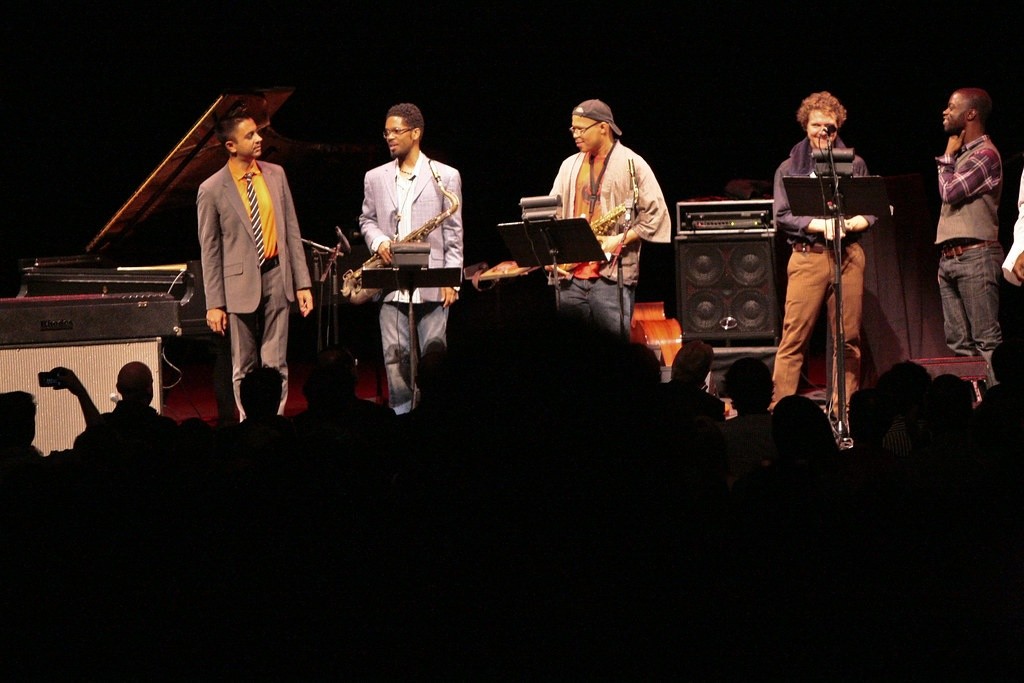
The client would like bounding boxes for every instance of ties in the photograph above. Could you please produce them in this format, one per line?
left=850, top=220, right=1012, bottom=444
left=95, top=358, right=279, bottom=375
left=244, top=171, right=266, bottom=269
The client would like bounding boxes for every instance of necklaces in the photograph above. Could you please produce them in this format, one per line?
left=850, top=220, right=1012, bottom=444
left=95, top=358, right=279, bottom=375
left=588, top=141, right=617, bottom=223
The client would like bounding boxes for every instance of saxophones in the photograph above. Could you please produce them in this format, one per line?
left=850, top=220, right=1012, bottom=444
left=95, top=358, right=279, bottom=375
left=543, top=158, right=639, bottom=274
left=339, top=173, right=460, bottom=307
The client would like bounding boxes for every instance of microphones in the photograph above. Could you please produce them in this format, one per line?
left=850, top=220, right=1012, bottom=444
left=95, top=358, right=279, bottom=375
left=822, top=125, right=837, bottom=136
left=625, top=190, right=635, bottom=222
left=335, top=225, right=354, bottom=254
left=109, top=393, right=120, bottom=404
left=0, top=336, right=163, bottom=457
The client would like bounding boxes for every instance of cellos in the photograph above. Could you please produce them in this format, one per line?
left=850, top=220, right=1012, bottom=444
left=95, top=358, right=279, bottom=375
left=629, top=300, right=731, bottom=418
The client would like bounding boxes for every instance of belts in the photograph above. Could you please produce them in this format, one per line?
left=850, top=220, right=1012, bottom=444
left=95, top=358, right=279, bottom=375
left=260, top=257, right=279, bottom=274
left=940, top=239, right=995, bottom=257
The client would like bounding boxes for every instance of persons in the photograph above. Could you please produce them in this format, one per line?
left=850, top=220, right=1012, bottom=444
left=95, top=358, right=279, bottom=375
left=359, top=105, right=463, bottom=415
left=218, top=340, right=472, bottom=682
left=197, top=106, right=313, bottom=422
left=0, top=367, right=104, bottom=683
left=694, top=394, right=843, bottom=683
left=934, top=88, right=1003, bottom=392
left=548, top=99, right=671, bottom=341
left=660, top=338, right=775, bottom=472
left=773, top=91, right=878, bottom=446
left=73, top=360, right=307, bottom=683
left=849, top=359, right=972, bottom=453
left=1002, top=169, right=1024, bottom=287
left=973, top=344, right=1024, bottom=415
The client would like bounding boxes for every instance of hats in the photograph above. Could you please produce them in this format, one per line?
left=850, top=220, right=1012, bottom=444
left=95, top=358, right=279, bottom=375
left=574, top=98, right=622, bottom=136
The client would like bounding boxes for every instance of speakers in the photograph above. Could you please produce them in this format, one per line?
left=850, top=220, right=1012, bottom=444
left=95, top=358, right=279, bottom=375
left=674, top=234, right=778, bottom=347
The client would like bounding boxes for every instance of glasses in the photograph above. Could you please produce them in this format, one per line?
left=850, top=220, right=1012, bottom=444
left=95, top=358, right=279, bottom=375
left=383, top=128, right=414, bottom=139
left=569, top=121, right=600, bottom=134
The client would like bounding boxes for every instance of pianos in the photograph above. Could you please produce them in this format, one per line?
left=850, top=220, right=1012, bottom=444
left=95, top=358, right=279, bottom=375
left=1, top=91, right=298, bottom=345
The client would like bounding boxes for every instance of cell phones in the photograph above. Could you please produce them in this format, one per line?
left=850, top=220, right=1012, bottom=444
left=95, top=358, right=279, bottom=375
left=38, top=371, right=67, bottom=387
